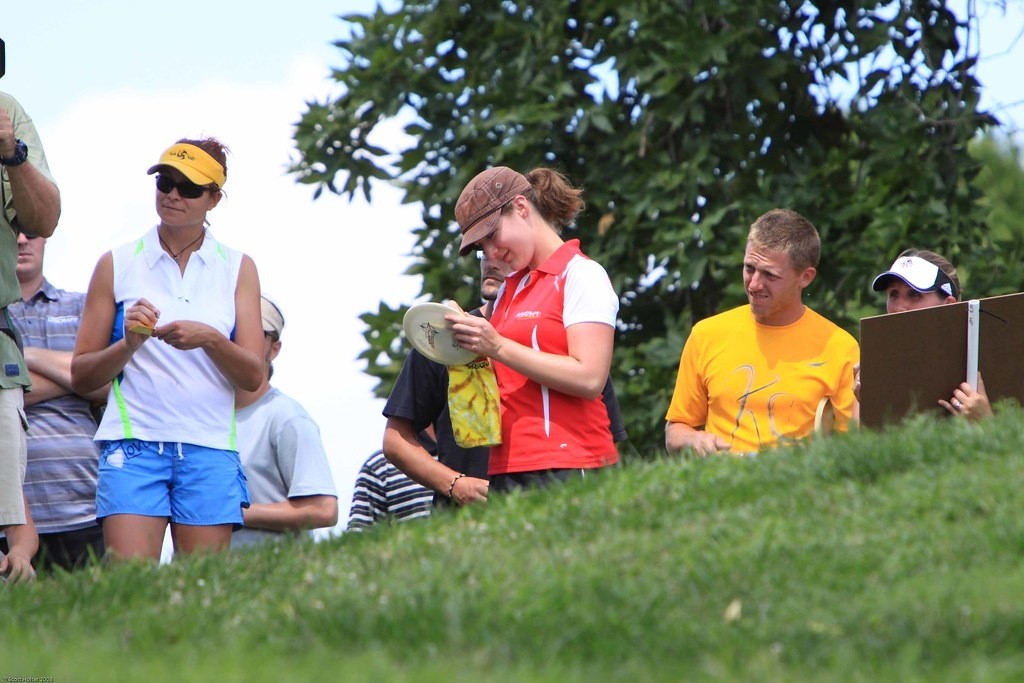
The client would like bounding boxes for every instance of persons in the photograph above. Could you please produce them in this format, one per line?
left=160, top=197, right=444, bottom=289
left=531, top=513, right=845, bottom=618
left=71, top=137, right=266, bottom=566
left=850, top=247, right=992, bottom=422
left=18, top=227, right=109, bottom=576
left=0, top=91, right=59, bottom=589
left=445, top=165, right=621, bottom=496
left=383, top=225, right=631, bottom=522
left=347, top=420, right=439, bottom=530
left=664, top=207, right=862, bottom=458
left=228, top=298, right=340, bottom=550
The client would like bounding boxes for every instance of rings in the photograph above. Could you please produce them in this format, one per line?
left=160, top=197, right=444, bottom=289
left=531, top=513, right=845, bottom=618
left=953, top=401, right=962, bottom=410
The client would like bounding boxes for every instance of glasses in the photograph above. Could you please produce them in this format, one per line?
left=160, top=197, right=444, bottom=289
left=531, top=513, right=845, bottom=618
left=476, top=251, right=487, bottom=259
left=155, top=175, right=215, bottom=198
left=9, top=223, right=38, bottom=239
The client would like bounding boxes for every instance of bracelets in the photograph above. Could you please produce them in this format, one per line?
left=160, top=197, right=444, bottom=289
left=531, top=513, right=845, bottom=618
left=448, top=473, right=468, bottom=500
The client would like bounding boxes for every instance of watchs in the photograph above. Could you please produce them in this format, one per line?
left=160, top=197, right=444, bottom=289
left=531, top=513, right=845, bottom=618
left=1, top=138, right=29, bottom=166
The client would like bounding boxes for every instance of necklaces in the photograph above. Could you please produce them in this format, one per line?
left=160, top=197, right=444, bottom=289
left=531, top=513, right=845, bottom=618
left=157, top=228, right=205, bottom=265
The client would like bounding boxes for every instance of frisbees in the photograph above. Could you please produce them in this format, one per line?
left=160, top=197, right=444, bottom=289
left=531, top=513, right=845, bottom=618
left=403, top=303, right=480, bottom=366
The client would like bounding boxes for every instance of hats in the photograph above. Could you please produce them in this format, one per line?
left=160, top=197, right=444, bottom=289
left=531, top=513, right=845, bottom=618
left=455, top=166, right=532, bottom=256
left=260, top=296, right=284, bottom=339
left=148, top=142, right=226, bottom=191
left=873, top=255, right=957, bottom=298
